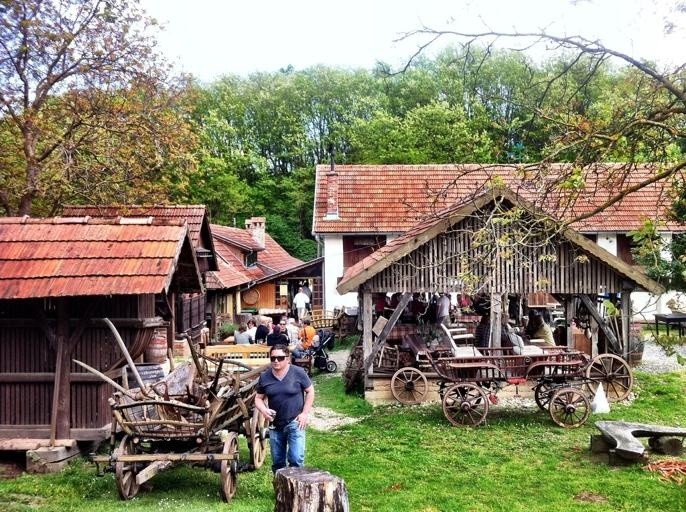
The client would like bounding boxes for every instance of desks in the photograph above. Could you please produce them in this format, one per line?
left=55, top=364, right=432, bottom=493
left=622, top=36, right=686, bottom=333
left=224, top=336, right=236, bottom=344
left=654, top=312, right=686, bottom=337
left=194, top=357, right=271, bottom=372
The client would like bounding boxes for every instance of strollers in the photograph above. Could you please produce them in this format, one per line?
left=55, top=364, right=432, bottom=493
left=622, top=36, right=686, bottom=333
left=297, top=327, right=337, bottom=376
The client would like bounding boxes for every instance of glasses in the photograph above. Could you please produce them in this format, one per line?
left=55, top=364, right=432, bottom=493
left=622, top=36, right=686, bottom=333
left=271, top=356, right=289, bottom=361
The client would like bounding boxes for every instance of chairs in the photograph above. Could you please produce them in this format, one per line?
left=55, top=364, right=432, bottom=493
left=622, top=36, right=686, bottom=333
left=404, top=324, right=549, bottom=368
left=294, top=344, right=314, bottom=376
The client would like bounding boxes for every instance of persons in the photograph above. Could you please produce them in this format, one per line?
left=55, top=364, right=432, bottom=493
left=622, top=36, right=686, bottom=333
left=376, top=292, right=451, bottom=324
left=234, top=318, right=320, bottom=357
left=293, top=284, right=312, bottom=321
left=476, top=312, right=508, bottom=348
left=256, top=344, right=315, bottom=474
left=526, top=310, right=556, bottom=346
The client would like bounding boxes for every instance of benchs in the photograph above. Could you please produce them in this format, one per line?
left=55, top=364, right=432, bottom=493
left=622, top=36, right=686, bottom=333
left=308, top=307, right=337, bottom=329
left=593, top=420, right=685, bottom=464
left=202, top=344, right=270, bottom=359
